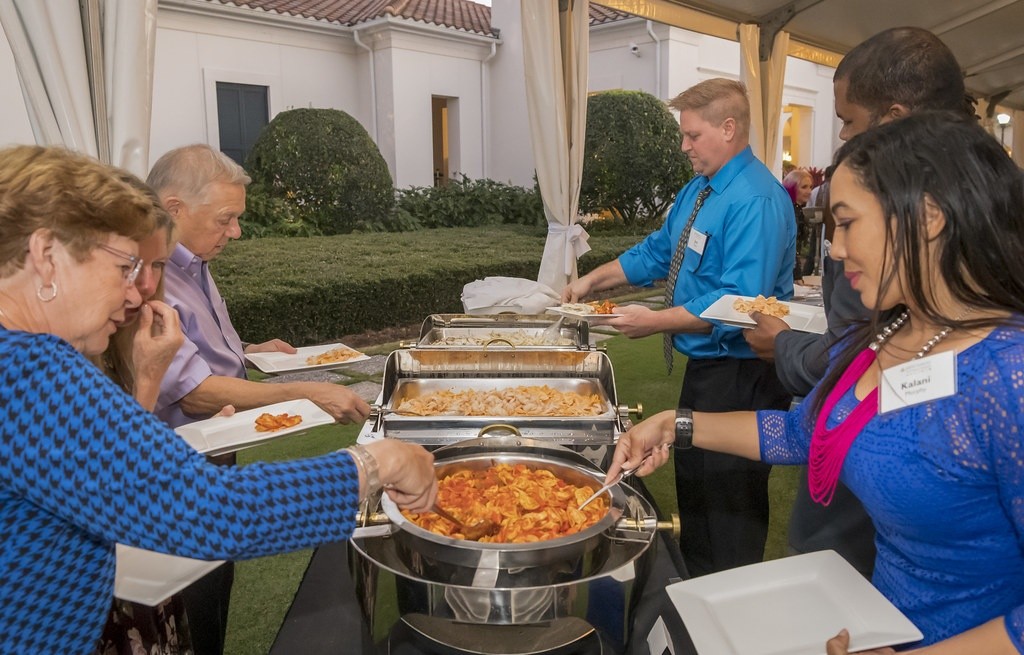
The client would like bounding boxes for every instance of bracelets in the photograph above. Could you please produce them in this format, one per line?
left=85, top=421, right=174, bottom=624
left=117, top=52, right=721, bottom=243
left=336, top=444, right=383, bottom=526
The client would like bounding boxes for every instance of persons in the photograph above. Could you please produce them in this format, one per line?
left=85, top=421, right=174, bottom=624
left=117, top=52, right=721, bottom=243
left=83, top=143, right=372, bottom=655
left=0, top=145, right=439, bottom=655
left=742, top=25, right=967, bottom=397
left=561, top=77, right=797, bottom=578
left=604, top=111, right=1024, bottom=655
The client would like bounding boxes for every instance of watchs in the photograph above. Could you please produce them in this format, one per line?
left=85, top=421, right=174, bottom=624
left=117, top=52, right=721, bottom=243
left=674, top=409, right=694, bottom=451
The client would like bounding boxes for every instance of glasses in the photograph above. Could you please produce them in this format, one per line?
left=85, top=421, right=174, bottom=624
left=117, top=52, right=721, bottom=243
left=26, top=227, right=144, bottom=287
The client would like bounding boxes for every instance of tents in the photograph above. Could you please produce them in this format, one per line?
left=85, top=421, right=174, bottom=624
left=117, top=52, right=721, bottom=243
left=0, top=1, right=1024, bottom=313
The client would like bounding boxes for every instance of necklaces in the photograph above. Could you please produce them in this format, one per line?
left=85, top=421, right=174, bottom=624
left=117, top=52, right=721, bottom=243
left=808, top=308, right=976, bottom=506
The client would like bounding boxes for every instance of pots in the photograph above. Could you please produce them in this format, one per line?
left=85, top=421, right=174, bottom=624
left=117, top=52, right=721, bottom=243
left=347, top=422, right=681, bottom=654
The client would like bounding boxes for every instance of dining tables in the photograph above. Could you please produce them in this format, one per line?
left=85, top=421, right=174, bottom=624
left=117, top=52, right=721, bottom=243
left=269, top=447, right=693, bottom=655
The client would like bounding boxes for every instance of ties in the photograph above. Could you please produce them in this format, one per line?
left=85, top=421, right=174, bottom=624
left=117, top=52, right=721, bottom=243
left=663, top=184, right=712, bottom=375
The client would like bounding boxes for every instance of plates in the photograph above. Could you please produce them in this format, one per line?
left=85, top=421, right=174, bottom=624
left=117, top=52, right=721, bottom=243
left=698, top=294, right=828, bottom=335
left=172, top=398, right=336, bottom=456
left=665, top=549, right=924, bottom=655
left=546, top=306, right=624, bottom=322
left=244, top=342, right=372, bottom=376
left=113, top=542, right=226, bottom=607
